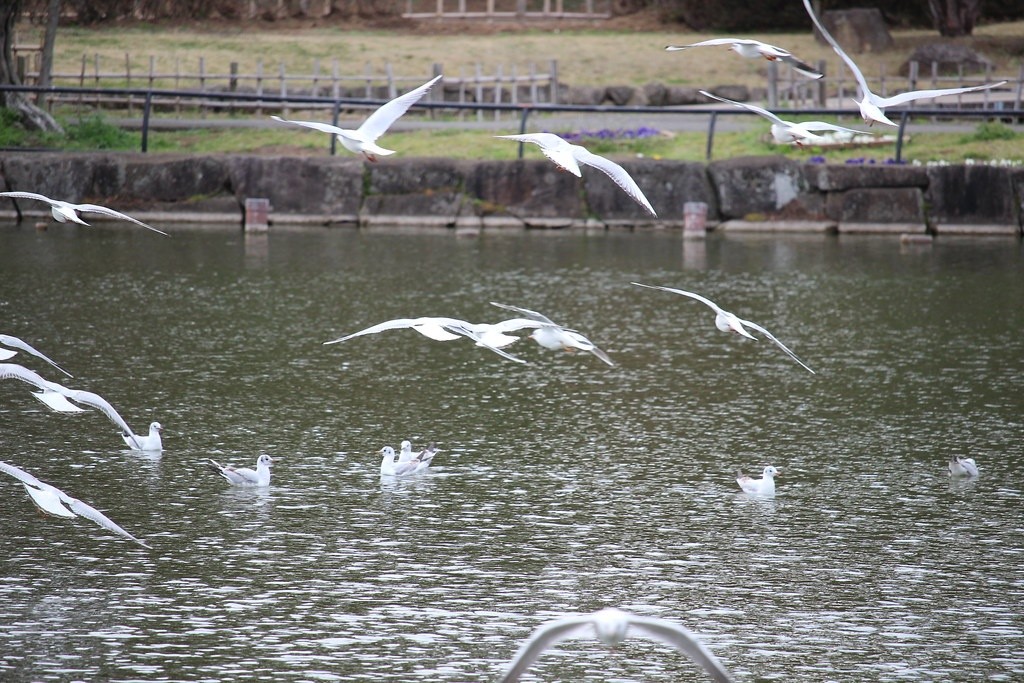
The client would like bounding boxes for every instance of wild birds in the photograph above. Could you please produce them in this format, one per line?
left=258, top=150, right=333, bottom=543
left=0, top=191, right=173, bottom=239
left=205, top=454, right=280, bottom=489
left=663, top=1, right=1009, bottom=149
left=494, top=607, right=742, bottom=683
left=269, top=75, right=444, bottom=164
left=374, top=440, right=437, bottom=477
left=0, top=332, right=164, bottom=549
left=321, top=301, right=615, bottom=374
left=491, top=132, right=659, bottom=221
left=948, top=456, right=978, bottom=478
left=630, top=282, right=816, bottom=376
left=734, top=465, right=781, bottom=498
